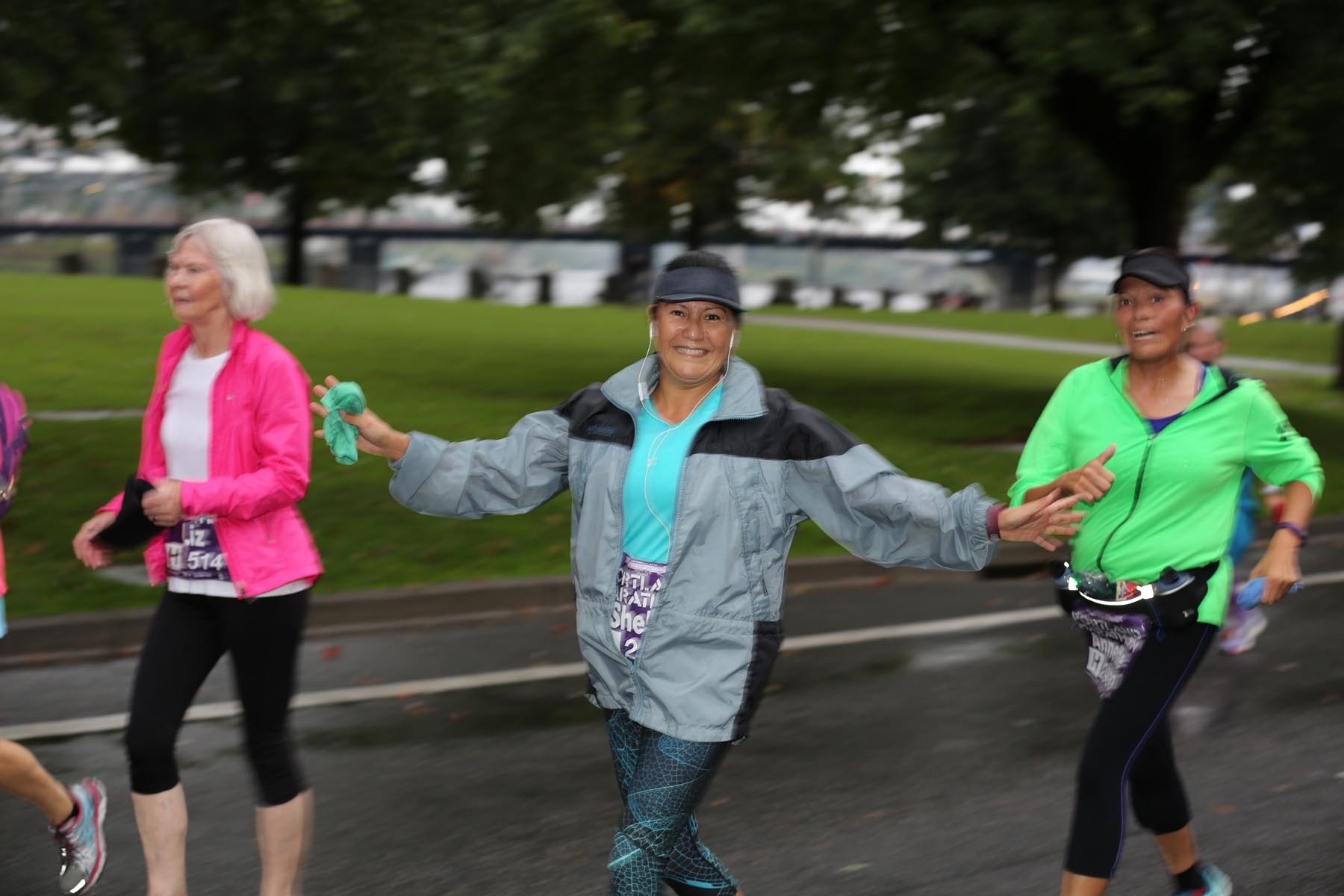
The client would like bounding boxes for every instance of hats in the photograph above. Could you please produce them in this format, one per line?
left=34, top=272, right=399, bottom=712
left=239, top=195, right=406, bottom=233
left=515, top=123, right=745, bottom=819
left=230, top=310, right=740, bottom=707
left=654, top=265, right=749, bottom=315
left=1106, top=251, right=1191, bottom=296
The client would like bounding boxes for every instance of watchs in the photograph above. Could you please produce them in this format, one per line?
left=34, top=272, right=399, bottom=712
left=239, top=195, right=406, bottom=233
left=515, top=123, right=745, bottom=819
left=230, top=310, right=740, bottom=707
left=1275, top=522, right=1309, bottom=549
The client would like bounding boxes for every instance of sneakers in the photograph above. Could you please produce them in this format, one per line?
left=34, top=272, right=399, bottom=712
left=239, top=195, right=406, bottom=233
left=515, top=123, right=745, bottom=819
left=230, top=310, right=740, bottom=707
left=48, top=775, right=109, bottom=896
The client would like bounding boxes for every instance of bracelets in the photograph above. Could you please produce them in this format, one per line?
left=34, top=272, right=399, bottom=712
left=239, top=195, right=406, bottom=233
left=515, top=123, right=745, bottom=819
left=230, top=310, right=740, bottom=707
left=986, top=502, right=1007, bottom=543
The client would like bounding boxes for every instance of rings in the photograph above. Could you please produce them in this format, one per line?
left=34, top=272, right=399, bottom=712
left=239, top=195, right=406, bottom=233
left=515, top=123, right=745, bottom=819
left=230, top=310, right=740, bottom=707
left=152, top=515, right=157, bottom=522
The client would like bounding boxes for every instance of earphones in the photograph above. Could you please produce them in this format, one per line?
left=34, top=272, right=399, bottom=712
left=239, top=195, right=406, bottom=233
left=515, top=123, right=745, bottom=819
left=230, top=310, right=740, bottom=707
left=730, top=332, right=735, bottom=348
left=649, top=322, right=655, bottom=340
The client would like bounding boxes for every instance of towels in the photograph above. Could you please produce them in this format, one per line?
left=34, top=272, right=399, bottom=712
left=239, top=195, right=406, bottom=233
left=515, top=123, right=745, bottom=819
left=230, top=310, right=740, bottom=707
left=318, top=379, right=366, bottom=465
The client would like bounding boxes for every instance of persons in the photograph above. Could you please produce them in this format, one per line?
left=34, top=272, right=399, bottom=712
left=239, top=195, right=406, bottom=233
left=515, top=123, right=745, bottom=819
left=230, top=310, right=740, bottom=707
left=71, top=219, right=324, bottom=896
left=1006, top=237, right=1327, bottom=896
left=309, top=249, right=1086, bottom=896
left=0, top=383, right=108, bottom=896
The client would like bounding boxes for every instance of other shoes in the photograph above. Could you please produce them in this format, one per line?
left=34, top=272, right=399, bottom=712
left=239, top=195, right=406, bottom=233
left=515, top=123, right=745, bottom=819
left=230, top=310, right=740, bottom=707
left=1218, top=602, right=1269, bottom=654
left=1172, top=863, right=1234, bottom=896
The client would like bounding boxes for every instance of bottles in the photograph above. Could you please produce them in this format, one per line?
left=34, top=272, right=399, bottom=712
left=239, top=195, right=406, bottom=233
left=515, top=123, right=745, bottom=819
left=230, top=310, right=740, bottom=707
left=1048, top=558, right=1075, bottom=587
left=1158, top=568, right=1192, bottom=592
